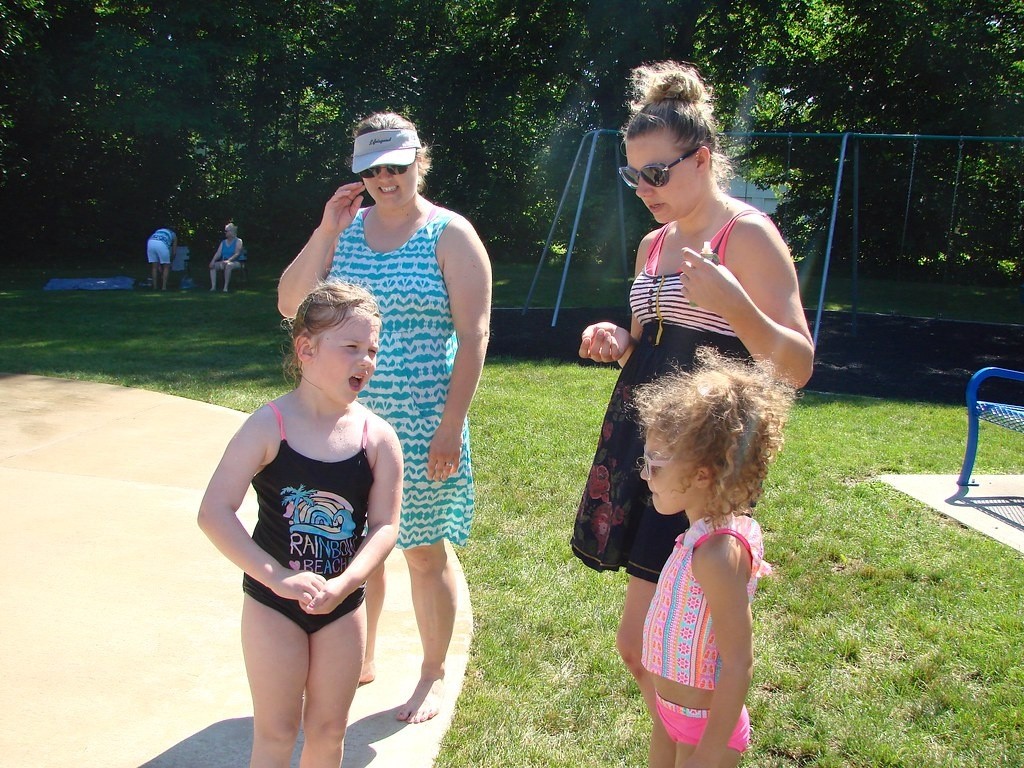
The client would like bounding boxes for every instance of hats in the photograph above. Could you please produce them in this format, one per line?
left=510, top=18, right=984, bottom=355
left=351, top=128, right=422, bottom=174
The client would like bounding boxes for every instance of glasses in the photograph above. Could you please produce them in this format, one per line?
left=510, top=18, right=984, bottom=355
left=359, top=165, right=409, bottom=178
left=617, top=145, right=713, bottom=189
left=224, top=230, right=231, bottom=234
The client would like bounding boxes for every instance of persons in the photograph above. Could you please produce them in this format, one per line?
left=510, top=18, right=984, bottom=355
left=630, top=347, right=794, bottom=768
left=148, top=227, right=178, bottom=291
left=277, top=112, right=493, bottom=723
left=197, top=281, right=405, bottom=768
left=576, top=62, right=814, bottom=723
left=208, top=222, right=243, bottom=294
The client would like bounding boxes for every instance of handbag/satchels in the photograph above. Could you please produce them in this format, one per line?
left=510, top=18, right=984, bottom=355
left=177, top=274, right=195, bottom=289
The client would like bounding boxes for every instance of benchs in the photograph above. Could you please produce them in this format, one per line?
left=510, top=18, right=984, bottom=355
left=955, top=367, right=1024, bottom=486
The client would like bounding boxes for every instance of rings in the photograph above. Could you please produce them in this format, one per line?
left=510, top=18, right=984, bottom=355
left=446, top=463, right=453, bottom=466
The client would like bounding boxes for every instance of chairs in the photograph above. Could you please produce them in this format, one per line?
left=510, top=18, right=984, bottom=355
left=215, top=247, right=247, bottom=293
